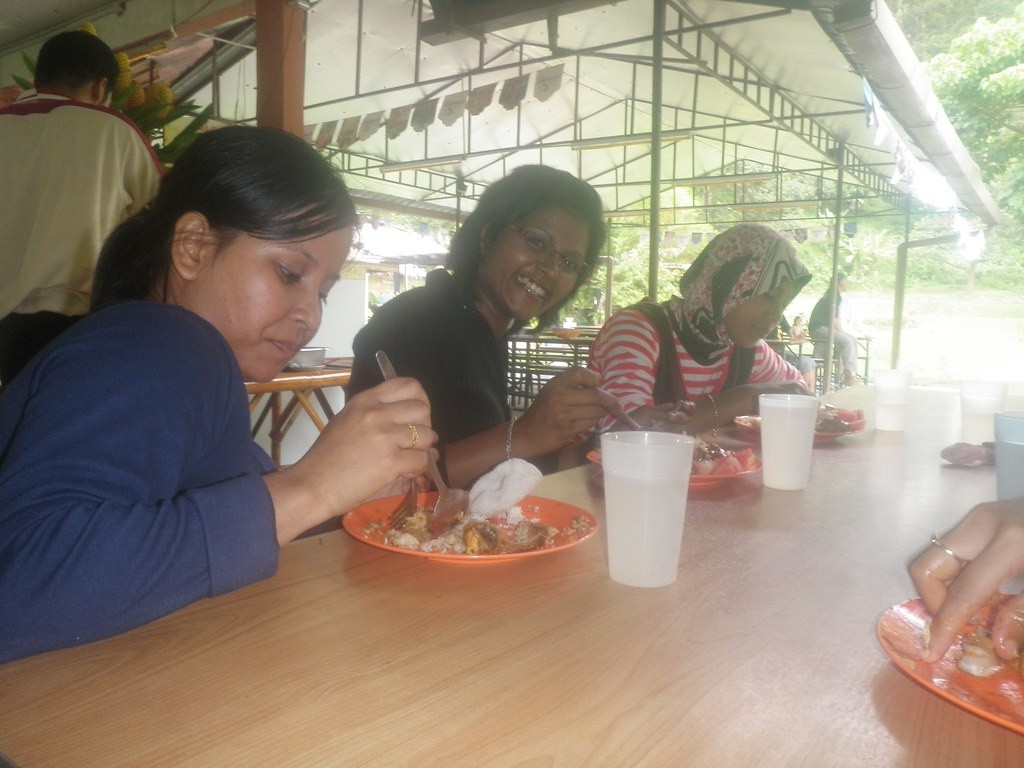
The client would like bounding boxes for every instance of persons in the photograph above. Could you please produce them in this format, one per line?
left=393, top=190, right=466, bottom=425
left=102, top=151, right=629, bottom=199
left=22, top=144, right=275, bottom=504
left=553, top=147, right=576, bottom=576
left=907, top=495, right=1024, bottom=664
left=564, top=224, right=812, bottom=464
left=1, top=29, right=167, bottom=395
left=808, top=271, right=862, bottom=386
left=766, top=315, right=819, bottom=393
left=344, top=164, right=621, bottom=493
left=0, top=126, right=440, bottom=666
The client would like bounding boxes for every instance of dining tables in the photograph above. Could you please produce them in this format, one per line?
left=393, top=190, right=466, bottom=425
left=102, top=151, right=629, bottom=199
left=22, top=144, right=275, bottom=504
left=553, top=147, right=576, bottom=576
left=0, top=386, right=1023, bottom=768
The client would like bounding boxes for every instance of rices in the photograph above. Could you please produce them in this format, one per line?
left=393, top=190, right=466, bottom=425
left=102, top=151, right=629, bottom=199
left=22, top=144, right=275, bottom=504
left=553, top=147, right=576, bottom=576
left=384, top=504, right=541, bottom=556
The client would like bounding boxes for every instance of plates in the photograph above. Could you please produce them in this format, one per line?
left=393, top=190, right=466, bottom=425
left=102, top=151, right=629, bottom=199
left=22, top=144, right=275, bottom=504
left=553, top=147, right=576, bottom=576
left=586, top=447, right=762, bottom=486
left=342, top=491, right=600, bottom=564
left=288, top=365, right=325, bottom=370
left=734, top=415, right=865, bottom=441
left=876, top=594, right=1024, bottom=735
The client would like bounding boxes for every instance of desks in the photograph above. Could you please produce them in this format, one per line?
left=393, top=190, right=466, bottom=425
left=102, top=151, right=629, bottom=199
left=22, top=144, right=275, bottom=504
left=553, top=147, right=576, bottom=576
left=506, top=326, right=828, bottom=410
left=243, top=357, right=353, bottom=465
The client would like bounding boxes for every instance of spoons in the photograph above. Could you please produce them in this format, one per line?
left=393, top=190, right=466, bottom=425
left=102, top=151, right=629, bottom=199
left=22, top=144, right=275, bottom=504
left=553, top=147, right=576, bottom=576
left=377, top=351, right=469, bottom=522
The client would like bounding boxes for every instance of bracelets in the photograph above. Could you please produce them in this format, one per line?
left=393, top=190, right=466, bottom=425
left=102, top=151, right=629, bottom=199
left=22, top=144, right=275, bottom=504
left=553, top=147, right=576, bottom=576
left=707, top=394, right=720, bottom=437
left=504, top=416, right=518, bottom=460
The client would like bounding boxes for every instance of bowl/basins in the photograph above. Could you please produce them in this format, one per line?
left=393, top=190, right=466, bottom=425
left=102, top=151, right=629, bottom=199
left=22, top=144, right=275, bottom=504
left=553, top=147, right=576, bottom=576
left=553, top=328, right=582, bottom=337
left=291, top=346, right=325, bottom=366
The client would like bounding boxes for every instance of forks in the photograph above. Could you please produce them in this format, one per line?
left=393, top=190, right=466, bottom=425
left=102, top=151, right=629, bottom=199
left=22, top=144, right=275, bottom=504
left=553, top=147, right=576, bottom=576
left=385, top=478, right=418, bottom=531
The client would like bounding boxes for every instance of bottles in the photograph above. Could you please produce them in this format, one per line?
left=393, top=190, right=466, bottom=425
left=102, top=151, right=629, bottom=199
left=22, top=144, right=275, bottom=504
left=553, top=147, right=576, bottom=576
left=775, top=325, right=782, bottom=340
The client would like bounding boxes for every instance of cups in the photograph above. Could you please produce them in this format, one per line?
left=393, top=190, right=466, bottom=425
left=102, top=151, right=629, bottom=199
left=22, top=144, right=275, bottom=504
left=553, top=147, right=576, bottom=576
left=994, top=412, right=1024, bottom=502
left=758, top=393, right=819, bottom=489
left=874, top=368, right=910, bottom=430
left=600, top=430, right=696, bottom=587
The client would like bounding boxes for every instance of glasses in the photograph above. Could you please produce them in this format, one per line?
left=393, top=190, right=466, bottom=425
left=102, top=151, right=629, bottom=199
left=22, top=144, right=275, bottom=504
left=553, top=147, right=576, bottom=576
left=507, top=221, right=594, bottom=280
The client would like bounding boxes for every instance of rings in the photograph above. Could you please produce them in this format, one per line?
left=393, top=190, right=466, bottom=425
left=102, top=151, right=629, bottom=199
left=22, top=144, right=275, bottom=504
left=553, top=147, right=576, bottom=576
left=931, top=536, right=962, bottom=566
left=408, top=423, right=418, bottom=449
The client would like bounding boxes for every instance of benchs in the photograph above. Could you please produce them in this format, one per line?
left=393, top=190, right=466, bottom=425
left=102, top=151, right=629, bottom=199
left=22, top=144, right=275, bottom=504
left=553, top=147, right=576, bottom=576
left=507, top=346, right=838, bottom=404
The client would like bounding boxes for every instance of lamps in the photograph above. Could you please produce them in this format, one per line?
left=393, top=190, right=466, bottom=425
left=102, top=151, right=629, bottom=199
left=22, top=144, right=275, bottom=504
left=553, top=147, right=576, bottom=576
left=571, top=131, right=691, bottom=151
left=730, top=201, right=821, bottom=210
left=379, top=155, right=467, bottom=172
left=603, top=210, right=649, bottom=217
left=671, top=173, right=777, bottom=187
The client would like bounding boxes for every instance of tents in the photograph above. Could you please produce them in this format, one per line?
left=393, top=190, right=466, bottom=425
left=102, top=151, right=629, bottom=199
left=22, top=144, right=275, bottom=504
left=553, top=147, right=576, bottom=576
left=162, top=0, right=1001, bottom=393
